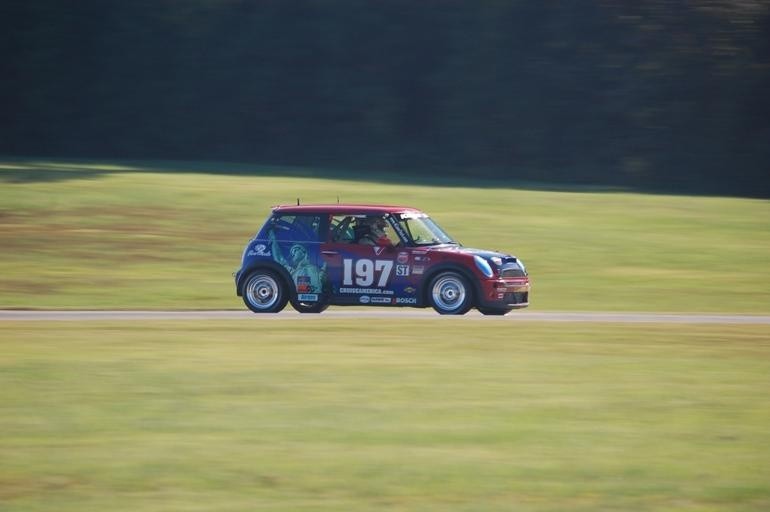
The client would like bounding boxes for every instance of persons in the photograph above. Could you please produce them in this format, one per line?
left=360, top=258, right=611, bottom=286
left=357, top=217, right=390, bottom=246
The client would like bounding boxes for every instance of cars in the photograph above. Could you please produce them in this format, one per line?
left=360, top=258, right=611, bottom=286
left=234, top=199, right=531, bottom=315
